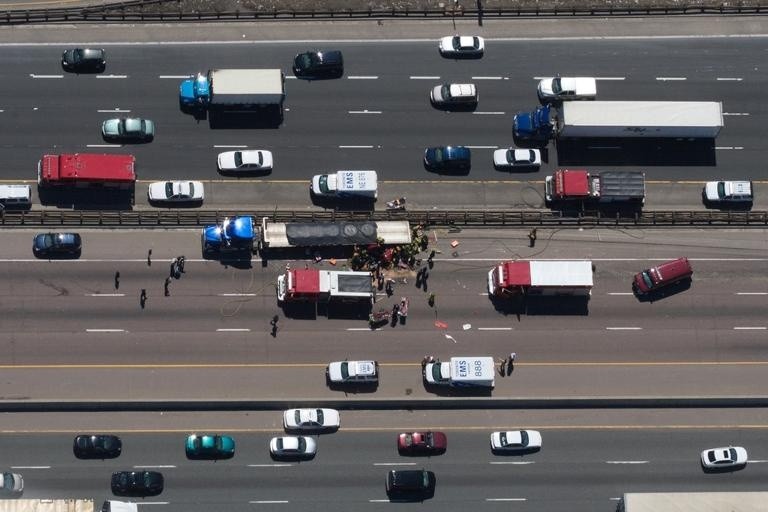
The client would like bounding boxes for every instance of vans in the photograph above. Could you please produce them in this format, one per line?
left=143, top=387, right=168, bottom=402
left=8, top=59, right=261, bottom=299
left=632, top=256, right=693, bottom=296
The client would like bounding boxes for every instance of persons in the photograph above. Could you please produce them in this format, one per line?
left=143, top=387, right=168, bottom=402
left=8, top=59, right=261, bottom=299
left=526, top=226, right=537, bottom=248
left=350, top=222, right=438, bottom=328
left=497, top=352, right=516, bottom=377
left=140, top=256, right=185, bottom=300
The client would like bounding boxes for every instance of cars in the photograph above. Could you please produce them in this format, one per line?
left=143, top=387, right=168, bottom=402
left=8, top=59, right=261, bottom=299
left=184, top=432, right=236, bottom=460
left=61, top=46, right=107, bottom=70
left=0, top=471, right=24, bottom=496
left=101, top=117, right=275, bottom=209
left=700, top=445, right=748, bottom=472
left=110, top=470, right=164, bottom=497
left=291, top=49, right=344, bottom=78
left=73, top=433, right=123, bottom=460
left=33, top=232, right=81, bottom=260
left=423, top=29, right=598, bottom=173
left=269, top=406, right=543, bottom=503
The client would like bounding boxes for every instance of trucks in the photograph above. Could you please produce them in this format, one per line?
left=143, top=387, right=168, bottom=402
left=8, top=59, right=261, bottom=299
left=0, top=182, right=33, bottom=206
left=545, top=168, right=646, bottom=205
left=200, top=213, right=412, bottom=255
left=487, top=259, right=594, bottom=299
left=277, top=269, right=378, bottom=307
left=37, top=152, right=137, bottom=192
left=310, top=169, right=380, bottom=201
left=178, top=67, right=286, bottom=111
left=422, top=357, right=496, bottom=390
left=0, top=499, right=138, bottom=512
left=511, top=99, right=726, bottom=144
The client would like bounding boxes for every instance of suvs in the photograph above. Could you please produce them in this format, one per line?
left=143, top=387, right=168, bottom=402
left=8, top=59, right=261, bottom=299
left=326, top=358, right=379, bottom=385
left=704, top=180, right=754, bottom=202
